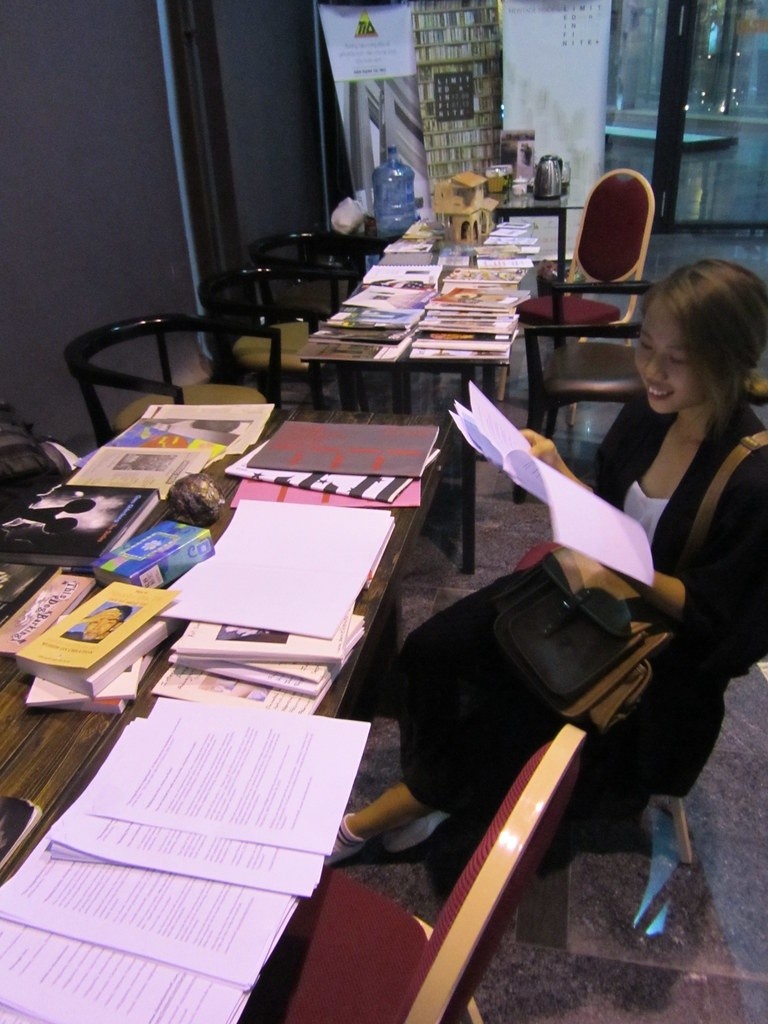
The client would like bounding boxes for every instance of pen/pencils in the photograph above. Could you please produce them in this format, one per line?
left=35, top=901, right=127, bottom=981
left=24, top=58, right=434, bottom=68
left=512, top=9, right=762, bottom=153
left=62, top=567, right=94, bottom=574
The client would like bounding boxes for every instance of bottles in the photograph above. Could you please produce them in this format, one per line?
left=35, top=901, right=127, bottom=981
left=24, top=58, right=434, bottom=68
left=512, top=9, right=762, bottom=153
left=372, top=144, right=416, bottom=236
left=561, top=161, right=572, bottom=184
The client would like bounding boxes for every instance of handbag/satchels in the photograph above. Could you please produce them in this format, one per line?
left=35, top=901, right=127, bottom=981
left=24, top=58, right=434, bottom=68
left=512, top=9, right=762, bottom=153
left=491, top=537, right=677, bottom=734
left=330, top=197, right=364, bottom=237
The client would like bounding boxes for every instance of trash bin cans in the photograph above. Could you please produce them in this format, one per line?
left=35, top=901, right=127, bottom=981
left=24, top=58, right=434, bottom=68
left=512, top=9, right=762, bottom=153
left=536, top=269, right=584, bottom=298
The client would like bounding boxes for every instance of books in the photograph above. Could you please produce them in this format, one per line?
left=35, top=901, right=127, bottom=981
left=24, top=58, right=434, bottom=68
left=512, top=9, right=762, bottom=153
left=1, top=404, right=441, bottom=1024
left=299, top=218, right=541, bottom=362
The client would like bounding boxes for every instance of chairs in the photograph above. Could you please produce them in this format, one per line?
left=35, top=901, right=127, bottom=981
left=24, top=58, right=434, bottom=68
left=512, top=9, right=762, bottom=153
left=196, top=229, right=402, bottom=420
left=496, top=167, right=654, bottom=425
left=512, top=341, right=646, bottom=505
left=238, top=722, right=588, bottom=1024
left=65, top=311, right=284, bottom=450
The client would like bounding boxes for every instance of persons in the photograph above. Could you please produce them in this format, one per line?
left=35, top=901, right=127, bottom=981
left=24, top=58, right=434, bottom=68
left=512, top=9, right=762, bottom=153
left=326, top=262, right=767, bottom=868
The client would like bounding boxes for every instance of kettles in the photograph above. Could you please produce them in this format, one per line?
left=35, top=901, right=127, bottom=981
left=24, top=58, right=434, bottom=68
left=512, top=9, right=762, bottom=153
left=533, top=154, right=564, bottom=200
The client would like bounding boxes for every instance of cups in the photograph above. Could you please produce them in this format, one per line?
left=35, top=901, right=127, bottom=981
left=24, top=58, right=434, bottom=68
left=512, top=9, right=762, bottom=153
left=512, top=176, right=527, bottom=195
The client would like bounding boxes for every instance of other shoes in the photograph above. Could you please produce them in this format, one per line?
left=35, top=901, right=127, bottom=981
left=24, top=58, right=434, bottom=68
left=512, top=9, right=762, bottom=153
left=323, top=843, right=363, bottom=867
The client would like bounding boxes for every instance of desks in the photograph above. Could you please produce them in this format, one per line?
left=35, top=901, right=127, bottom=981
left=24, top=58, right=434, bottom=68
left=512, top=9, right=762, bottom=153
left=495, top=173, right=587, bottom=286
left=0, top=411, right=454, bottom=883
left=299, top=235, right=522, bottom=572
